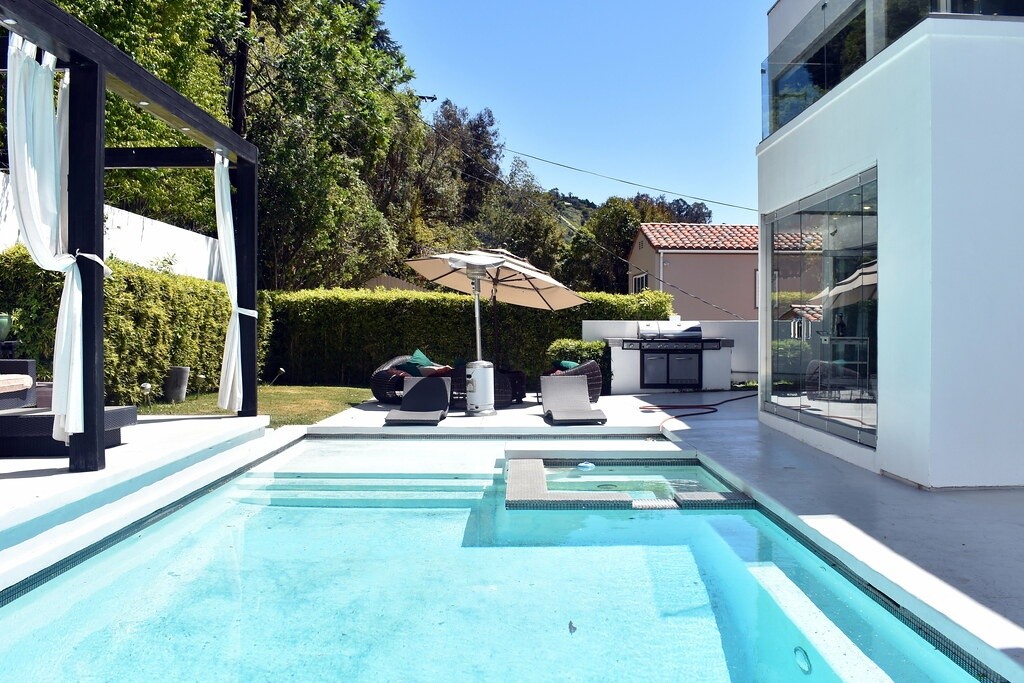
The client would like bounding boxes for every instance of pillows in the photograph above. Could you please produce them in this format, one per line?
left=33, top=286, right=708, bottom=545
left=551, top=360, right=580, bottom=371
left=395, top=348, right=434, bottom=377
left=386, top=369, right=413, bottom=377
left=419, top=364, right=454, bottom=377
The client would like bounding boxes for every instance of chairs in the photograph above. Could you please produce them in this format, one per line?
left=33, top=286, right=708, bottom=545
left=539, top=375, right=607, bottom=424
left=384, top=376, right=452, bottom=425
left=0, top=359, right=36, bottom=410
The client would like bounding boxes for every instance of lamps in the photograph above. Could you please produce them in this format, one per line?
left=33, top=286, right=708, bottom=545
left=277, top=367, right=285, bottom=375
left=196, top=374, right=204, bottom=383
left=139, top=383, right=151, bottom=395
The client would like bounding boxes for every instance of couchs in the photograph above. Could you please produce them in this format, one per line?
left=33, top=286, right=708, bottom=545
left=805, top=360, right=868, bottom=400
left=543, top=360, right=602, bottom=402
left=370, top=355, right=455, bottom=405
left=501, top=369, right=527, bottom=404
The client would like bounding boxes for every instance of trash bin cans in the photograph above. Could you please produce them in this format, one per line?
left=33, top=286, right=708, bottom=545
left=162, top=366, right=191, bottom=402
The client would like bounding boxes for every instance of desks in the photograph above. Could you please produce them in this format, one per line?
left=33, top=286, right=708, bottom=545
left=0, top=406, right=137, bottom=458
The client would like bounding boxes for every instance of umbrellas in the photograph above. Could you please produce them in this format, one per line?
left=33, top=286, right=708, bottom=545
left=403, top=248, right=592, bottom=371
left=806, top=259, right=877, bottom=308
